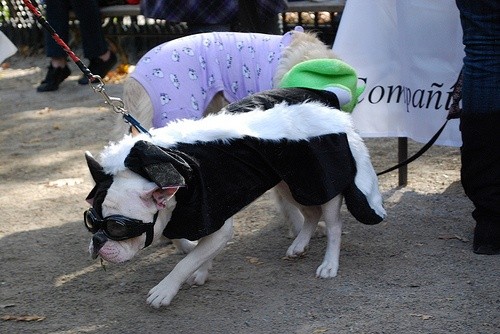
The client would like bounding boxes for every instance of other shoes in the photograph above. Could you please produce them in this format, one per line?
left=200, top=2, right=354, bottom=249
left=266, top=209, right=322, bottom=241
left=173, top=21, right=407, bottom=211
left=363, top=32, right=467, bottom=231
left=472, top=217, right=500, bottom=254
left=77, top=50, right=117, bottom=85
left=36, top=60, right=71, bottom=92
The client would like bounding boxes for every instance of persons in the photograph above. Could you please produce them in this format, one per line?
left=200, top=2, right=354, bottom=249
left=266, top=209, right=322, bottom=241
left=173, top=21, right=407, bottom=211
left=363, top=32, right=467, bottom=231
left=448, top=0, right=500, bottom=255
left=36, top=0, right=117, bottom=92
left=188, top=0, right=288, bottom=35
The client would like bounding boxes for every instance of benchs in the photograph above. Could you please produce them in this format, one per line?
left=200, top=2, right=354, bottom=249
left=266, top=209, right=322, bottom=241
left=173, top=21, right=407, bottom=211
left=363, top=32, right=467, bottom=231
left=103, top=1, right=345, bottom=59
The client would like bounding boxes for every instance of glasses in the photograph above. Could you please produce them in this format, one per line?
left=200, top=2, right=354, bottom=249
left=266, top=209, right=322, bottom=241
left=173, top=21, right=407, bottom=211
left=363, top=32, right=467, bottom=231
left=83, top=208, right=152, bottom=240
